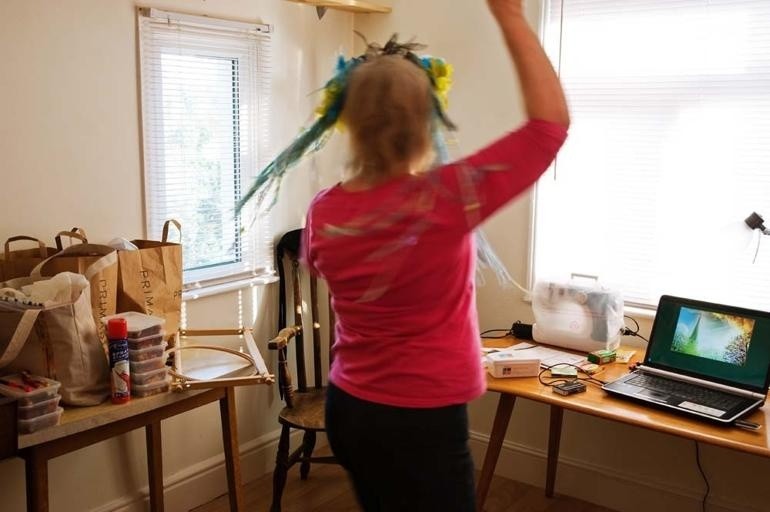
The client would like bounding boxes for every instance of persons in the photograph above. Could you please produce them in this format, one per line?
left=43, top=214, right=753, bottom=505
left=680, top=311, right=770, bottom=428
left=299, top=0, right=573, bottom=511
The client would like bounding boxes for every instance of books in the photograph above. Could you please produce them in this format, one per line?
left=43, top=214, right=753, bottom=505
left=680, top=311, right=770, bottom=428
left=498, top=341, right=587, bottom=372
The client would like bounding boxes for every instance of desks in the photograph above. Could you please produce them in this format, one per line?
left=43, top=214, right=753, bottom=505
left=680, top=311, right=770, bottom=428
left=8, top=348, right=264, bottom=510
left=474, top=333, right=768, bottom=508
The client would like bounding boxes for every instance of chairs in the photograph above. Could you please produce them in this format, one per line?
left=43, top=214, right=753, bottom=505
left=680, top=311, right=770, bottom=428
left=264, top=231, right=349, bottom=511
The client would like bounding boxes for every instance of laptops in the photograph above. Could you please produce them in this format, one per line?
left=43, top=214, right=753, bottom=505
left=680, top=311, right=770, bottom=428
left=602, top=295, right=770, bottom=425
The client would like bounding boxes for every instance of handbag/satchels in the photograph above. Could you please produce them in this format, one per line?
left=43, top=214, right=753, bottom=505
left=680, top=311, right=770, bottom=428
left=532, top=273, right=623, bottom=352
left=0, top=228, right=118, bottom=407
left=119, top=220, right=182, bottom=340
